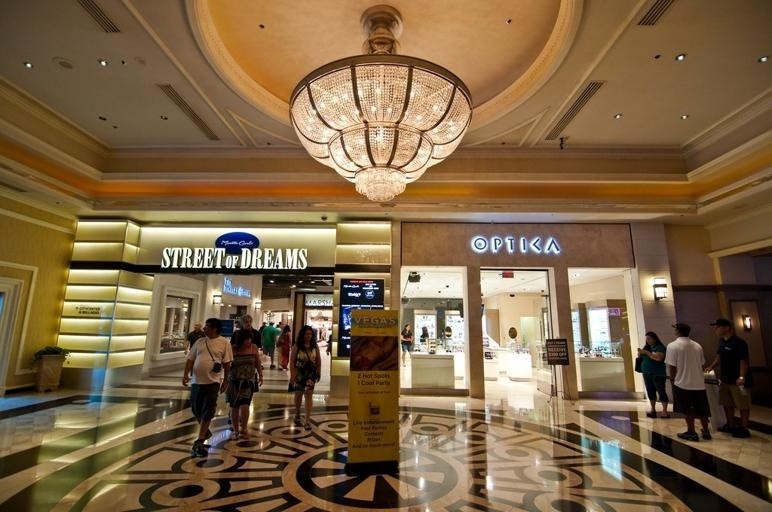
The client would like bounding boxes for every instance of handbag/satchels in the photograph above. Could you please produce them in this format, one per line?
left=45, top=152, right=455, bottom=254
left=212, top=363, right=221, bottom=373
left=635, top=358, right=643, bottom=372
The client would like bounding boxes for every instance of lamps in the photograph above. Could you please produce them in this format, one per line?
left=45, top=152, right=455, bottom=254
left=212, top=291, right=223, bottom=306
left=286, top=3, right=479, bottom=209
left=652, top=276, right=668, bottom=302
left=742, top=313, right=753, bottom=333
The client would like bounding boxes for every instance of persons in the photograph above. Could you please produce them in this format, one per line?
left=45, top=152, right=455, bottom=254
left=703, top=318, right=751, bottom=438
left=665, top=321, right=711, bottom=442
left=637, top=331, right=668, bottom=418
left=181, top=315, right=431, bottom=458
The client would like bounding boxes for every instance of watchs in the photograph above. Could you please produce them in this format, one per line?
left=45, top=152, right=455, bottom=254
left=739, top=375, right=743, bottom=379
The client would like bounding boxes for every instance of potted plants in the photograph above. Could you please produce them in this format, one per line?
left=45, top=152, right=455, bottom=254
left=26, top=344, right=73, bottom=394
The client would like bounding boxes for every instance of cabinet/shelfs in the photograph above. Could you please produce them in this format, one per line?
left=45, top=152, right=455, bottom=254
left=402, top=337, right=630, bottom=395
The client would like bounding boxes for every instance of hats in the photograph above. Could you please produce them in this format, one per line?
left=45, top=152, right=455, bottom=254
left=709, top=319, right=730, bottom=326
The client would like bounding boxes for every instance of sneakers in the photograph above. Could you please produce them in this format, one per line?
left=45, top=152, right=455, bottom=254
left=701, top=429, right=711, bottom=440
left=717, top=424, right=751, bottom=438
left=294, top=415, right=301, bottom=424
left=192, top=429, right=212, bottom=457
left=677, top=432, right=699, bottom=441
left=304, top=423, right=312, bottom=430
left=228, top=425, right=250, bottom=440
